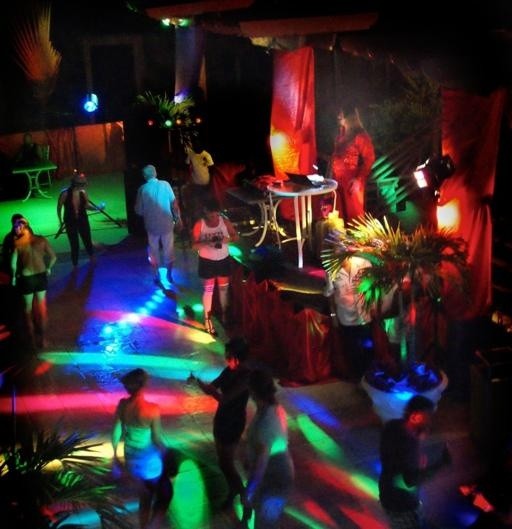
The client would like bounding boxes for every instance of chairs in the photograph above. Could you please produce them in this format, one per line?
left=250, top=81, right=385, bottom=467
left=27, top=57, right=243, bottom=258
left=36, top=144, right=54, bottom=185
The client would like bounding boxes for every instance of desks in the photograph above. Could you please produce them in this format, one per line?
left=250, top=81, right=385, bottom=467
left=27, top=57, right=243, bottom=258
left=269, top=178, right=338, bottom=269
left=227, top=186, right=293, bottom=247
left=12, top=160, right=58, bottom=202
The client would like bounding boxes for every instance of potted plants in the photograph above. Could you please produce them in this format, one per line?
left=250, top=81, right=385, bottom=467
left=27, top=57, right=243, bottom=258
left=320, top=212, right=474, bottom=430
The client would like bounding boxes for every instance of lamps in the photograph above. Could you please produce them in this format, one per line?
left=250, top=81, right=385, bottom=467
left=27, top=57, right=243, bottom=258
left=414, top=154, right=457, bottom=188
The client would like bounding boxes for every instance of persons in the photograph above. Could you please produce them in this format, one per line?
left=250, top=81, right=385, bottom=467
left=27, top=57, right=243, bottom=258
left=1, top=133, right=97, bottom=351
left=109, top=366, right=177, bottom=529
left=328, top=103, right=377, bottom=230
left=132, top=137, right=511, bottom=528
